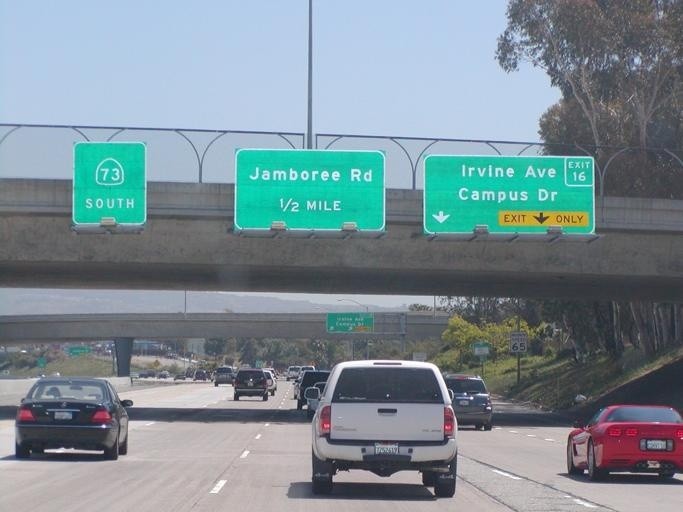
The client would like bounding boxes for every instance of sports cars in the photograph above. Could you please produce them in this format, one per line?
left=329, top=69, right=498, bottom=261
left=566, top=405, right=683, bottom=479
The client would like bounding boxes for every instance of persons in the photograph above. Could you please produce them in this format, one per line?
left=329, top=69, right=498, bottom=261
left=50, top=386, right=61, bottom=397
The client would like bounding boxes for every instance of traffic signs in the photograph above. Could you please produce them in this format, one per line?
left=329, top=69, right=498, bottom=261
left=74, top=142, right=146, bottom=224
left=424, top=156, right=596, bottom=237
left=236, top=147, right=385, bottom=232
left=326, top=313, right=372, bottom=334
left=510, top=332, right=528, bottom=353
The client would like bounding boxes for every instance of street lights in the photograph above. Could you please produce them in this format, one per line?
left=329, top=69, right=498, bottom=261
left=336, top=298, right=368, bottom=312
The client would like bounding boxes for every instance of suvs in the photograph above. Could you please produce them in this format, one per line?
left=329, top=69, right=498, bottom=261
left=442, top=374, right=491, bottom=430
left=304, top=359, right=458, bottom=496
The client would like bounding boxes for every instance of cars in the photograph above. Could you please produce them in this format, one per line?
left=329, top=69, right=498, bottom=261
left=14, top=377, right=133, bottom=461
left=130, top=365, right=329, bottom=409
